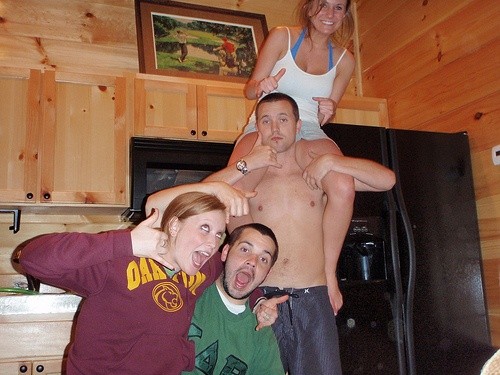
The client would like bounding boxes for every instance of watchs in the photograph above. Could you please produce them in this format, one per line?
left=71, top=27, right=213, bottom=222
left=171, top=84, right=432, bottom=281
left=236, top=159, right=248, bottom=175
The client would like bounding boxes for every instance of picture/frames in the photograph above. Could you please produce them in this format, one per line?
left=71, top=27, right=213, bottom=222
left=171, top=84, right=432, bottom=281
left=134, top=0, right=270, bottom=83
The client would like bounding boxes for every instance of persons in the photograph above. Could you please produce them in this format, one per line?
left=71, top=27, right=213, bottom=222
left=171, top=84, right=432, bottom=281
left=18, top=191, right=289, bottom=375
left=220, top=0, right=356, bottom=316
left=203, top=92, right=397, bottom=375
left=145, top=181, right=284, bottom=375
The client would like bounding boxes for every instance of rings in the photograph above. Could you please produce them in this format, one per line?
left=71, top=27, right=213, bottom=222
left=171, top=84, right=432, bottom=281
left=162, top=239, right=167, bottom=248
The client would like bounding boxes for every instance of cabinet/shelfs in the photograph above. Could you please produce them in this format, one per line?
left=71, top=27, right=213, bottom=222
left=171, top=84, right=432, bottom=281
left=0, top=65, right=131, bottom=214
left=328, top=95, right=389, bottom=129
left=130, top=72, right=261, bottom=144
left=0, top=311, right=77, bottom=375
